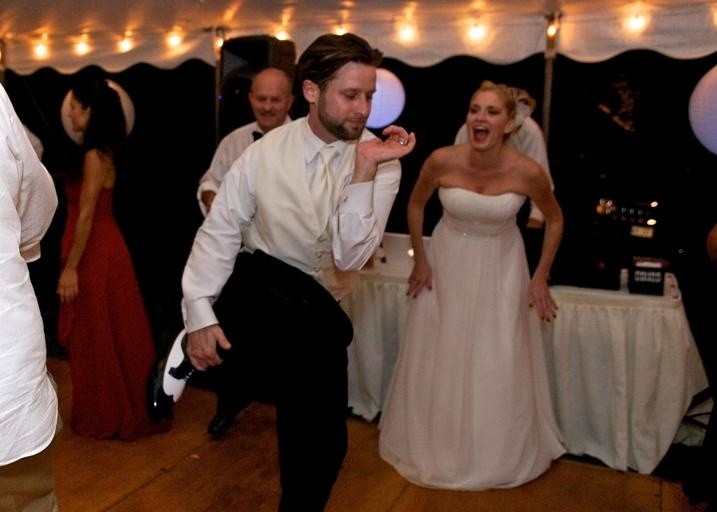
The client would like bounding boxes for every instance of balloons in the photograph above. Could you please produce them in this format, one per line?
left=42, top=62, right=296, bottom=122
left=364, top=65, right=408, bottom=128
left=684, top=65, right=716, bottom=150
left=59, top=74, right=136, bottom=148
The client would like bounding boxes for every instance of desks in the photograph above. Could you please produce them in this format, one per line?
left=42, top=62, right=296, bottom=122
left=334, top=232, right=710, bottom=474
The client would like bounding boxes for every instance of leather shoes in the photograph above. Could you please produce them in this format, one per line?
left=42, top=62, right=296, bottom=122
left=144, top=325, right=195, bottom=419
left=206, top=397, right=247, bottom=437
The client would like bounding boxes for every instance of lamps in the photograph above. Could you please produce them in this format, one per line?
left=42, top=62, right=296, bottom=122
left=546, top=11, right=565, bottom=38
left=206, top=26, right=230, bottom=47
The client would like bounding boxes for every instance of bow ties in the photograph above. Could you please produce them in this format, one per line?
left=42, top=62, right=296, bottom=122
left=251, top=130, right=264, bottom=142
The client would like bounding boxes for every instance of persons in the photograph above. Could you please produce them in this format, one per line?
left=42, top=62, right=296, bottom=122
left=455, top=107, right=554, bottom=258
left=379, top=83, right=564, bottom=490
left=194, top=68, right=293, bottom=436
left=148, top=32, right=417, bottom=512
left=56, top=67, right=171, bottom=442
left=0, top=81, right=61, bottom=512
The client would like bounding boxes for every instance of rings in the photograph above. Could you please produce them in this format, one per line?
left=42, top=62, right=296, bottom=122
left=397, top=141, right=404, bottom=144
